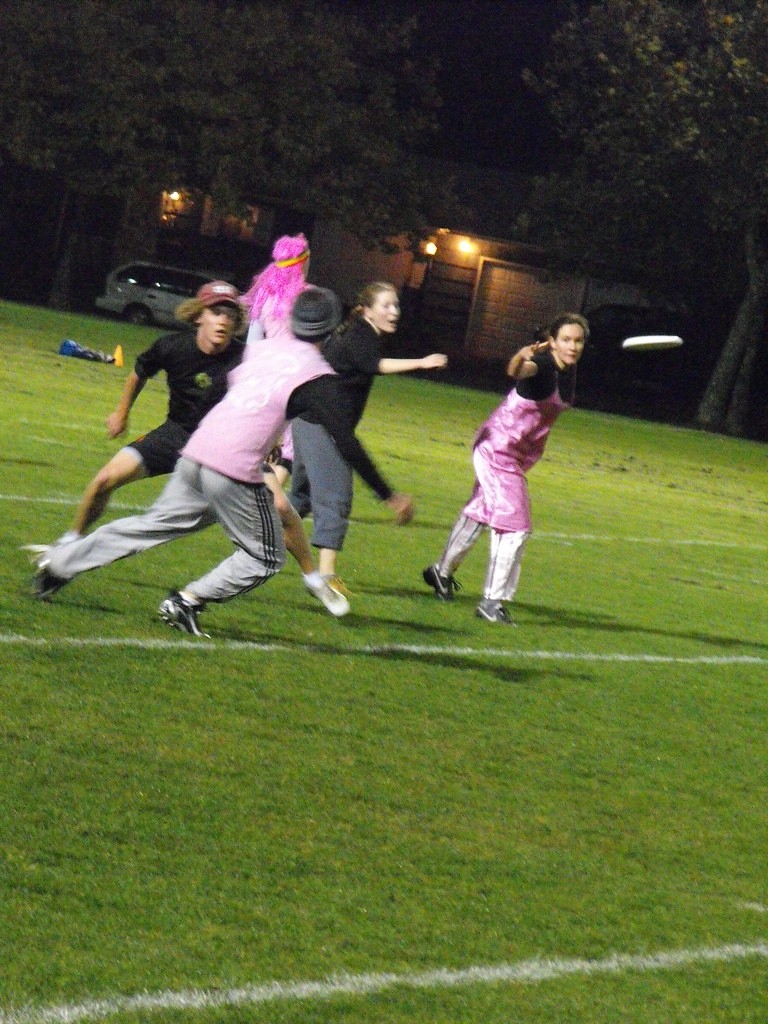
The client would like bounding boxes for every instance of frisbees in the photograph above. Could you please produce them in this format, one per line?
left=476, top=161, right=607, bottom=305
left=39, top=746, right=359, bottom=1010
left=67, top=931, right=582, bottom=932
left=623, top=334, right=681, bottom=350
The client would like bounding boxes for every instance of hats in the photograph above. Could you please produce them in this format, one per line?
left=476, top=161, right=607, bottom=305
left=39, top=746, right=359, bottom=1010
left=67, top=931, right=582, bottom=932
left=291, top=287, right=341, bottom=341
left=197, top=280, right=239, bottom=308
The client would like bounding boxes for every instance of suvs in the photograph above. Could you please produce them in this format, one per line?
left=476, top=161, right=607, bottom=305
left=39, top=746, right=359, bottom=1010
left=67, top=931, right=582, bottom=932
left=93, top=260, right=250, bottom=337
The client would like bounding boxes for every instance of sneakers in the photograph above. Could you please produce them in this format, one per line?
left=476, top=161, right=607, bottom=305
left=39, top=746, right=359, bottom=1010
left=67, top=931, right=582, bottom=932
left=33, top=565, right=68, bottom=604
left=159, top=590, right=211, bottom=639
left=421, top=563, right=464, bottom=602
left=476, top=597, right=515, bottom=625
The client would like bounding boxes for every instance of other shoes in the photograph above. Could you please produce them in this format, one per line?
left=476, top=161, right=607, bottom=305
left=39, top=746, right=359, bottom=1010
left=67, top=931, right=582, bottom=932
left=57, top=530, right=83, bottom=547
left=303, top=577, right=350, bottom=617
left=317, top=573, right=352, bottom=596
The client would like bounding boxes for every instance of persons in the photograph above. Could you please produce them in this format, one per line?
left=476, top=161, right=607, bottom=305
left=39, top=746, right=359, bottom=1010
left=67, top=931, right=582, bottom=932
left=18, top=280, right=352, bottom=616
left=29, top=286, right=415, bottom=639
left=237, top=235, right=311, bottom=487
left=423, top=313, right=589, bottom=626
left=286, top=282, right=448, bottom=601
